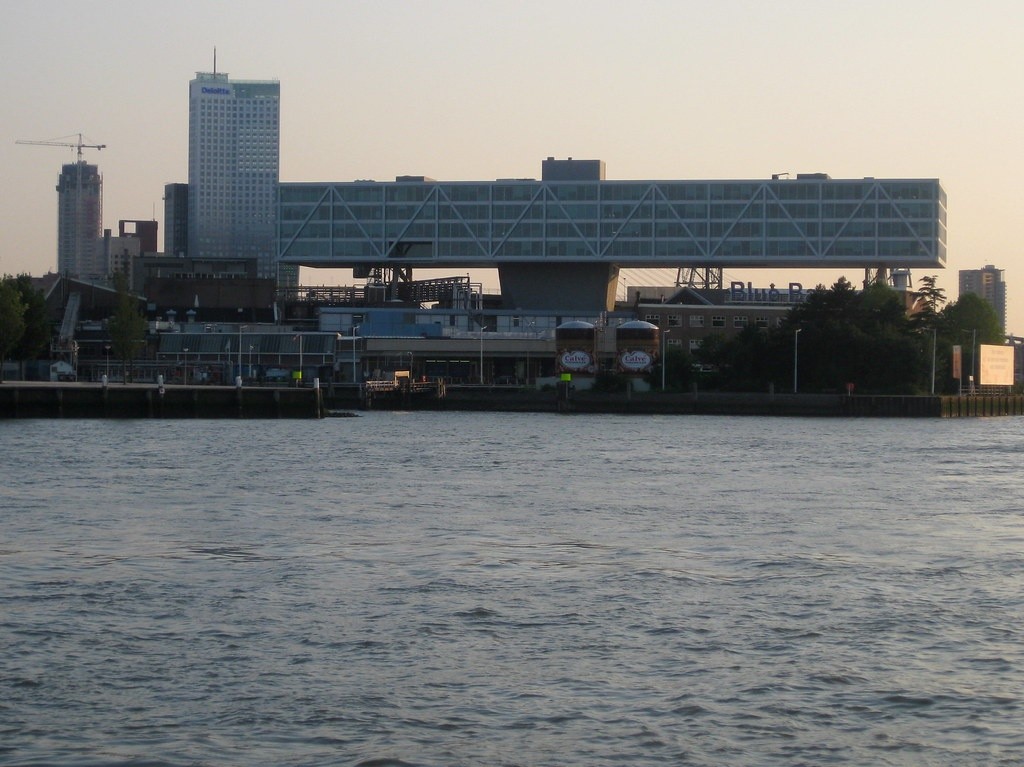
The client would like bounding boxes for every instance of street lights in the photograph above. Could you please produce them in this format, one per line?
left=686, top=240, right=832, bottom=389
left=794, top=328, right=802, bottom=393
left=526, top=321, right=534, bottom=386
left=352, top=326, right=360, bottom=383
left=407, top=351, right=413, bottom=383
left=480, top=326, right=488, bottom=385
left=662, top=329, right=671, bottom=392
left=238, top=324, right=247, bottom=376
left=103, top=341, right=112, bottom=384
left=961, top=328, right=977, bottom=395
left=921, top=327, right=937, bottom=397
left=181, top=343, right=190, bottom=385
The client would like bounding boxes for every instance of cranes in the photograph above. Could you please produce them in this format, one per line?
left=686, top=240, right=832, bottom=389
left=14, top=132, right=107, bottom=281
